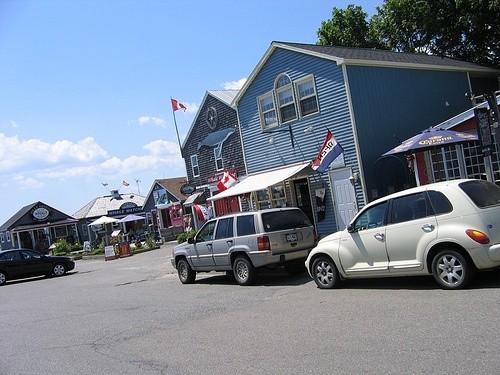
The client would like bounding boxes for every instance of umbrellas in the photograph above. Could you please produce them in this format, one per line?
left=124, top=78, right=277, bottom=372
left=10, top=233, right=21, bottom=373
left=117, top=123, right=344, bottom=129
left=87, top=216, right=120, bottom=227
left=116, top=214, right=147, bottom=224
left=377, top=127, right=478, bottom=162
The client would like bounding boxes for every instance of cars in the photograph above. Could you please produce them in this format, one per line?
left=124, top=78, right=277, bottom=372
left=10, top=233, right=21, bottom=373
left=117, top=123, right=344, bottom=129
left=305, top=177, right=496, bottom=291
left=0, top=248, right=76, bottom=286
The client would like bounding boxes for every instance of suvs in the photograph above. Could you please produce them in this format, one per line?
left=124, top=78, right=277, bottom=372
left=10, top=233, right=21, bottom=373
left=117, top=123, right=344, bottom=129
left=170, top=205, right=318, bottom=288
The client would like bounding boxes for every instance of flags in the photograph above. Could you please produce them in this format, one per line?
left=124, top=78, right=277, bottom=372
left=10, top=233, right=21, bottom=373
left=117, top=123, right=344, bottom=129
left=217, top=172, right=237, bottom=192
left=194, top=204, right=212, bottom=222
left=103, top=179, right=141, bottom=186
left=171, top=99, right=186, bottom=112
left=311, top=131, right=343, bottom=174
